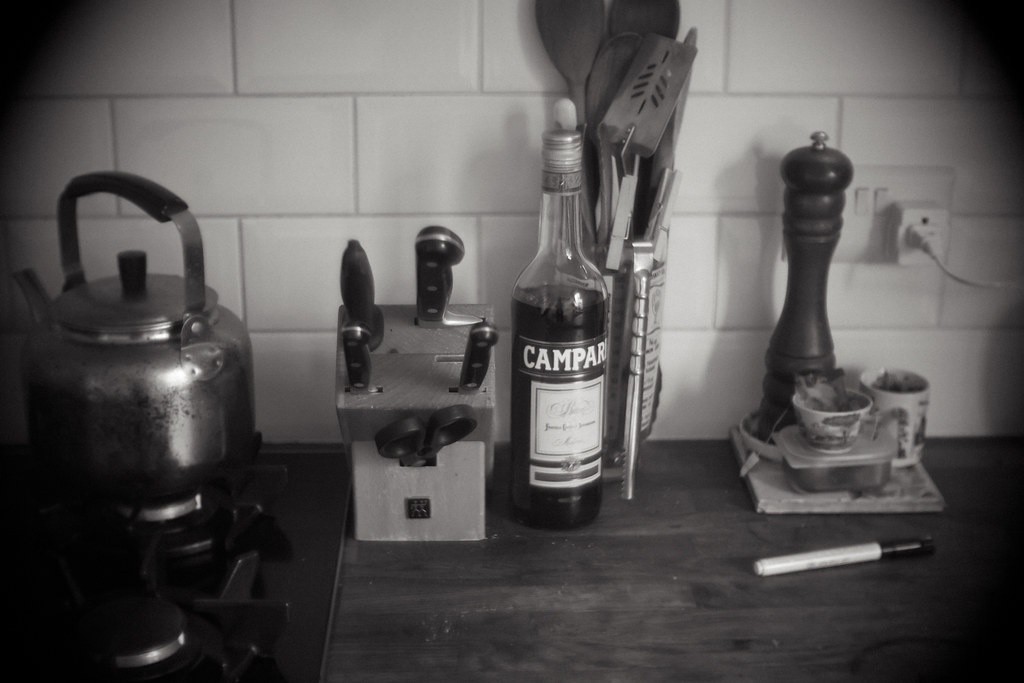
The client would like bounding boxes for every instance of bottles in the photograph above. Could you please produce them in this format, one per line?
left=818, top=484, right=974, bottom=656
left=507, top=93, right=614, bottom=531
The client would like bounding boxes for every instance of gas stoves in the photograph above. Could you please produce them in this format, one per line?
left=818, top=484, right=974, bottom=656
left=1, top=442, right=355, bottom=683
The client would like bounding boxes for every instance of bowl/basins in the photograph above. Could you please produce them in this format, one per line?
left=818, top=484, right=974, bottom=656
left=774, top=421, right=896, bottom=493
left=794, top=384, right=873, bottom=454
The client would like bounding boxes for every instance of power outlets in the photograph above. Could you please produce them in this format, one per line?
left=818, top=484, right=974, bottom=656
left=884, top=200, right=950, bottom=265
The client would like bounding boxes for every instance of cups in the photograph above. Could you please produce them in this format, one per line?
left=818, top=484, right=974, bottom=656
left=860, top=365, right=933, bottom=467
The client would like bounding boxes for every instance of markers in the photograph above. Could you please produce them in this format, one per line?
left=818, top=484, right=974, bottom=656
left=752, top=535, right=938, bottom=578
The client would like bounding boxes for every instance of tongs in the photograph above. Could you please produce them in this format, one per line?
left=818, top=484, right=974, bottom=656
left=605, top=235, right=654, bottom=503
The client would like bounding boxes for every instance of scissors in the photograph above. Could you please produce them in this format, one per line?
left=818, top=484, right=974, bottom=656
left=373, top=403, right=478, bottom=467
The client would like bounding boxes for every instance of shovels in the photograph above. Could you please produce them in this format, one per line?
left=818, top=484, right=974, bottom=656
left=534, top=0, right=606, bottom=133
left=596, top=32, right=700, bottom=269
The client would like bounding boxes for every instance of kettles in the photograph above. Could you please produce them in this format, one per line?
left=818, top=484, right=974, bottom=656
left=11, top=166, right=263, bottom=512
